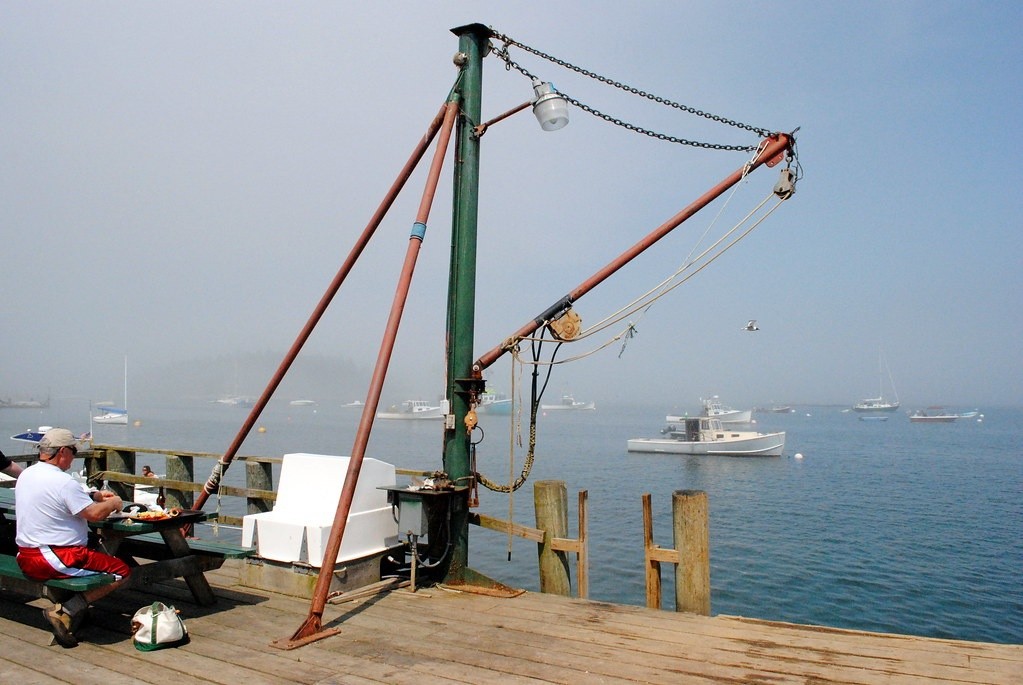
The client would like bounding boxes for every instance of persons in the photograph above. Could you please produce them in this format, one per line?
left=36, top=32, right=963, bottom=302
left=0, top=452, right=24, bottom=558
left=142, top=466, right=155, bottom=479
left=14, top=428, right=129, bottom=648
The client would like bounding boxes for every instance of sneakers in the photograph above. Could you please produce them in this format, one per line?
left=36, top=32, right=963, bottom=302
left=42, top=602, right=81, bottom=648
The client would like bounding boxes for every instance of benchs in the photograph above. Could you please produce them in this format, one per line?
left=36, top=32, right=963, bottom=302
left=3, top=512, right=257, bottom=570
left=0, top=554, right=116, bottom=617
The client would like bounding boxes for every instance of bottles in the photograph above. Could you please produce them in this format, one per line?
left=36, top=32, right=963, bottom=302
left=157, top=486, right=165, bottom=511
left=99, top=479, right=118, bottom=497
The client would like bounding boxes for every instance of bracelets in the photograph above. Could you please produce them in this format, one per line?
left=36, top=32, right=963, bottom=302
left=88, top=492, right=95, bottom=501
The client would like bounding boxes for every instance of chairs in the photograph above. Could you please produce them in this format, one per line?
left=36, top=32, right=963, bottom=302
left=242, top=453, right=398, bottom=569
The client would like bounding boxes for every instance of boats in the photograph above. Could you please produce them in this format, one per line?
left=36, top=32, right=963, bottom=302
left=772, top=406, right=790, bottom=413
left=909, top=405, right=958, bottom=423
left=472, top=382, right=517, bottom=417
left=342, top=400, right=365, bottom=408
left=10, top=426, right=91, bottom=444
left=627, top=418, right=786, bottom=458
left=290, top=400, right=314, bottom=405
left=1, top=387, right=51, bottom=409
left=377, top=400, right=445, bottom=420
left=541, top=395, right=594, bottom=410
left=81, top=473, right=167, bottom=489
left=954, top=411, right=978, bottom=420
left=667, top=396, right=751, bottom=424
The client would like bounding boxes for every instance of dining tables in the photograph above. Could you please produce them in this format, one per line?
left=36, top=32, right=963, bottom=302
left=0, top=488, right=220, bottom=610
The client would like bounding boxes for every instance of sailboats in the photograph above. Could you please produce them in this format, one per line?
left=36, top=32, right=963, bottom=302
left=852, top=347, right=900, bottom=412
left=93, top=358, right=129, bottom=425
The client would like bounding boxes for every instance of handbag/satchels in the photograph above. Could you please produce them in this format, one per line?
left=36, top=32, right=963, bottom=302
left=127, top=601, right=192, bottom=652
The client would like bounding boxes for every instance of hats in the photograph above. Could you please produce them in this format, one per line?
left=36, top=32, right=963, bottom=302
left=36, top=428, right=87, bottom=451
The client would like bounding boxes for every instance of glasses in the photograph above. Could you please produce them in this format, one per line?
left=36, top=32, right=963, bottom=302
left=65, top=445, right=78, bottom=455
